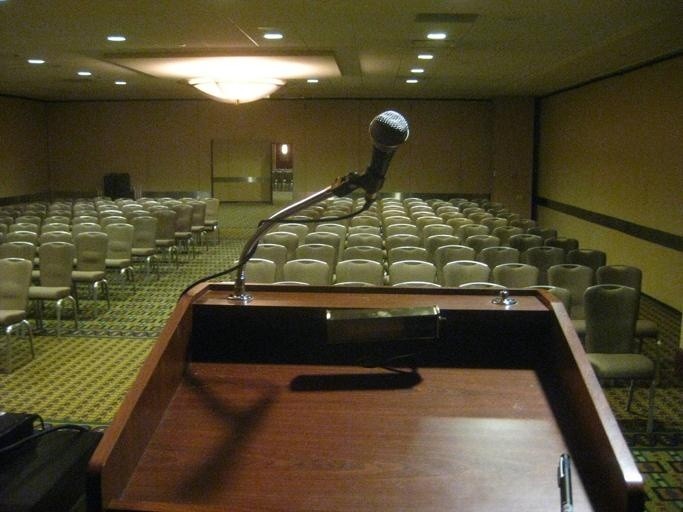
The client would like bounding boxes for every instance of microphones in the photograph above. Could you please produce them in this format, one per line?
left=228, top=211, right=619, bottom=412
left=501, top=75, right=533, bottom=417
left=364, top=110, right=410, bottom=205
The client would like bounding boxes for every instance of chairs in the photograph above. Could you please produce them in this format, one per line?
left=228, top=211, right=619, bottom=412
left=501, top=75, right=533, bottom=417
left=583, top=284, right=657, bottom=433
left=0, top=189, right=221, bottom=363
left=596, top=265, right=660, bottom=360
left=233, top=192, right=609, bottom=338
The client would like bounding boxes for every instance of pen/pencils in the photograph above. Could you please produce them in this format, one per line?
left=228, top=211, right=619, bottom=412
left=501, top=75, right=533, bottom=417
left=559, top=453, right=573, bottom=512
